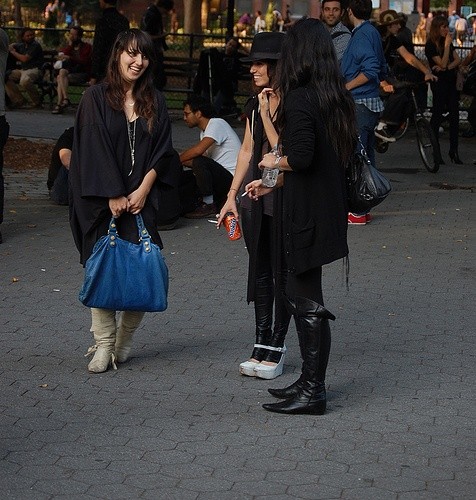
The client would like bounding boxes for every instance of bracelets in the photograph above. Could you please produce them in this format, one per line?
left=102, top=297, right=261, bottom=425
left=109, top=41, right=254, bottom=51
left=276, top=156, right=283, bottom=172
left=230, top=187, right=238, bottom=193
left=446, top=66, right=449, bottom=71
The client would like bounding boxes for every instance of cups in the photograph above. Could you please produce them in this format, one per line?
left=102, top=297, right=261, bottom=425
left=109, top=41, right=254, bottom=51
left=448, top=32, right=453, bottom=39
left=58, top=52, right=64, bottom=61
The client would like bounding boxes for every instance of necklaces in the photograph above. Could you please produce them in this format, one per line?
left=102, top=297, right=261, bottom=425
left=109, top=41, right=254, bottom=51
left=124, top=102, right=136, bottom=177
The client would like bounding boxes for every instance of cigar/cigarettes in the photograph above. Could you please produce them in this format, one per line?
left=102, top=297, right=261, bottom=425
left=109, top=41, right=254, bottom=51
left=208, top=220, right=218, bottom=224
left=242, top=188, right=252, bottom=197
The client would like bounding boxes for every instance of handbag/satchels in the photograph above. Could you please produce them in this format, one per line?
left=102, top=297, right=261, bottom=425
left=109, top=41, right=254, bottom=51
left=79, top=211, right=168, bottom=311
left=344, top=149, right=393, bottom=209
left=465, top=59, right=476, bottom=93
left=456, top=71, right=464, bottom=90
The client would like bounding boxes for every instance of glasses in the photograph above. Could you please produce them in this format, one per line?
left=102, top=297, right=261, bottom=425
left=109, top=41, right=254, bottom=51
left=182, top=111, right=196, bottom=116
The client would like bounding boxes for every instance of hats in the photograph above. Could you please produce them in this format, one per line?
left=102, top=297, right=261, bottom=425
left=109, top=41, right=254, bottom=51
left=239, top=32, right=284, bottom=62
left=377, top=11, right=404, bottom=25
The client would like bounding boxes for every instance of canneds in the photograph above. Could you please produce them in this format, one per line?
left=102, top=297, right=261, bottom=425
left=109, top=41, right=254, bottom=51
left=223, top=213, right=242, bottom=240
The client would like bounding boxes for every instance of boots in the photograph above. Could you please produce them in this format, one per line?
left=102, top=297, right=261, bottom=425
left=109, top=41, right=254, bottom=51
left=261, top=290, right=334, bottom=414
left=268, top=314, right=331, bottom=399
left=87, top=307, right=118, bottom=373
left=115, top=311, right=147, bottom=363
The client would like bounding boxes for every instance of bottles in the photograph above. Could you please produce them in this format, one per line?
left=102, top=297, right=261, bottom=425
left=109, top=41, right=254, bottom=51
left=262, top=144, right=281, bottom=188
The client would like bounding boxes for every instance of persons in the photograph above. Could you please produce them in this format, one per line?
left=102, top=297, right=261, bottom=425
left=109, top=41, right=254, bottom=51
left=67, top=27, right=173, bottom=371
left=217, top=33, right=293, bottom=379
left=244, top=19, right=357, bottom=414
left=0, top=0, right=476, bottom=225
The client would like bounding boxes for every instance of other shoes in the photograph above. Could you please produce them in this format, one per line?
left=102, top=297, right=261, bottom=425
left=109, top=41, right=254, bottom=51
left=9, top=99, right=28, bottom=107
left=184, top=202, right=219, bottom=218
left=31, top=102, right=40, bottom=108
left=366, top=211, right=373, bottom=222
left=374, top=127, right=396, bottom=142
left=348, top=213, right=367, bottom=226
left=157, top=224, right=176, bottom=231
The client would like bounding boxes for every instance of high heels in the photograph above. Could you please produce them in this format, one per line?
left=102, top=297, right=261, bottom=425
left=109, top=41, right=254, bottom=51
left=254, top=343, right=288, bottom=379
left=433, top=154, right=446, bottom=165
left=449, top=149, right=464, bottom=164
left=238, top=343, right=265, bottom=377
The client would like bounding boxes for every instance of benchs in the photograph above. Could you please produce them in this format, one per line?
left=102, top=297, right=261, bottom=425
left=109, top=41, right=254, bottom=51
left=14, top=49, right=256, bottom=111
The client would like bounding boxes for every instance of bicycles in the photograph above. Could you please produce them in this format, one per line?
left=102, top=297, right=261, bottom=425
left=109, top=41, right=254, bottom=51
left=372, top=76, right=441, bottom=173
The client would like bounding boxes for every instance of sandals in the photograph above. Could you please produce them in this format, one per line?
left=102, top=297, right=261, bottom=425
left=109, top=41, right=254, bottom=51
left=61, top=98, right=72, bottom=107
left=52, top=105, right=64, bottom=115
